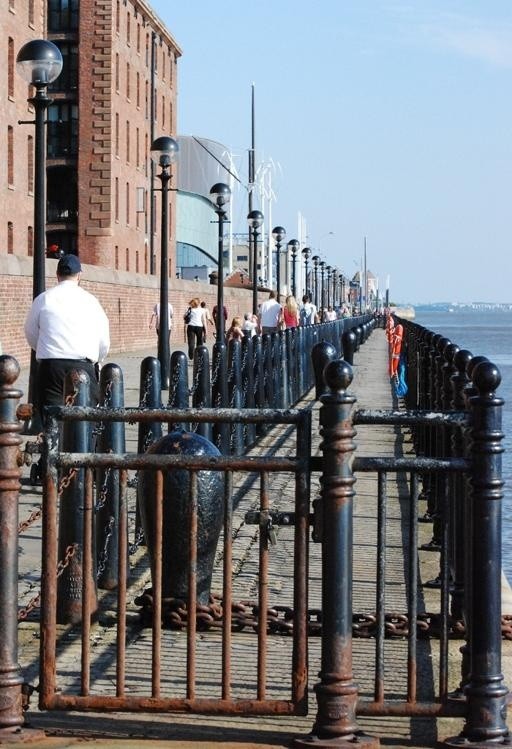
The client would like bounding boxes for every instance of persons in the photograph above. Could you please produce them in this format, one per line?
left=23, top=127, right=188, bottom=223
left=23, top=253, right=111, bottom=478
left=183, top=291, right=359, bottom=359
left=149, top=303, right=174, bottom=361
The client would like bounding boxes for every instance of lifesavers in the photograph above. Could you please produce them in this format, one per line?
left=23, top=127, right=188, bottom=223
left=387, top=315, right=395, bottom=345
left=389, top=325, right=403, bottom=374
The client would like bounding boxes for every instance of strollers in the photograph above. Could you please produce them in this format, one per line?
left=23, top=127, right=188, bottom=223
left=29, top=362, right=99, bottom=487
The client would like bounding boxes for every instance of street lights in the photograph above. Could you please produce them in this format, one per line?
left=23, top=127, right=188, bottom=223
left=246, top=210, right=264, bottom=316
left=287, top=239, right=301, bottom=302
left=15, top=41, right=64, bottom=435
left=150, top=136, right=179, bottom=391
left=317, top=230, right=335, bottom=256
left=210, top=183, right=231, bottom=346
left=302, top=248, right=360, bottom=324
left=271, top=225, right=286, bottom=305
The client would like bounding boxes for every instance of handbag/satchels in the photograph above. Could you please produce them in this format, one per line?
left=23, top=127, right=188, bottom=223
left=184, top=307, right=191, bottom=324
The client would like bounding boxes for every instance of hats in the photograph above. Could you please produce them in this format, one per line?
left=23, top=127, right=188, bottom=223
left=57, top=253, right=82, bottom=275
